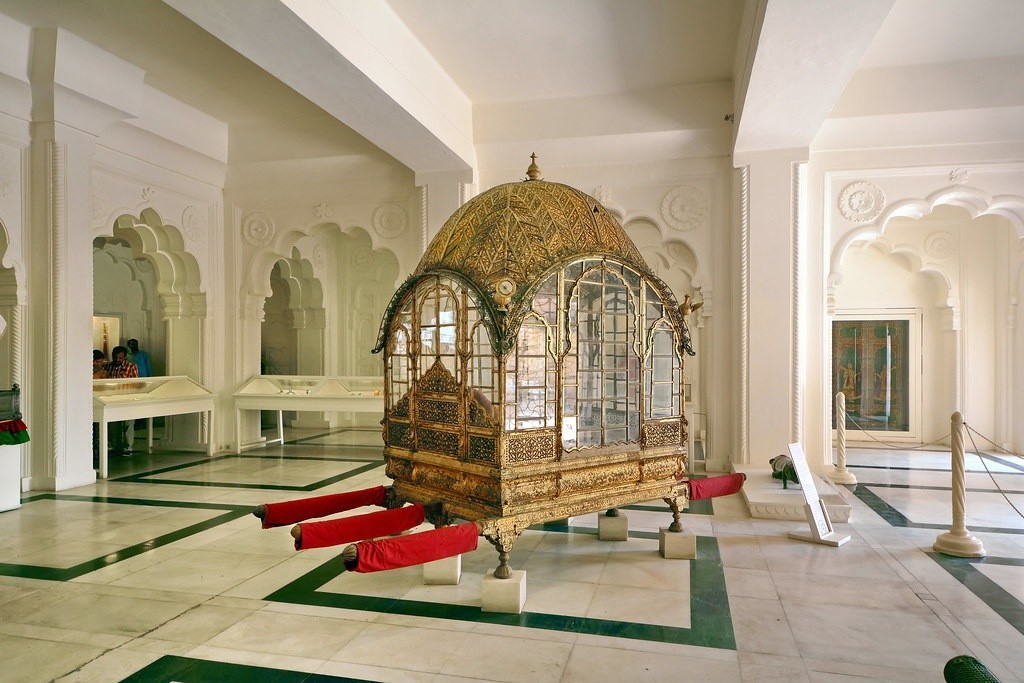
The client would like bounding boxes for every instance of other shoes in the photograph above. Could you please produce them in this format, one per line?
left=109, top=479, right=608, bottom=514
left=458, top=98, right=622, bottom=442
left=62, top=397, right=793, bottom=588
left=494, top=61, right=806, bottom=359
left=123, top=451, right=132, bottom=456
left=108, top=449, right=124, bottom=457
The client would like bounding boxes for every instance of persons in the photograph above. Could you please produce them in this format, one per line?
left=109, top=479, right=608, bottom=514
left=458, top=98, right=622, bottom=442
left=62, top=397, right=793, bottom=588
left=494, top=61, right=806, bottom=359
left=124, top=339, right=152, bottom=431
left=93, top=346, right=139, bottom=456
left=261, top=351, right=265, bottom=428
